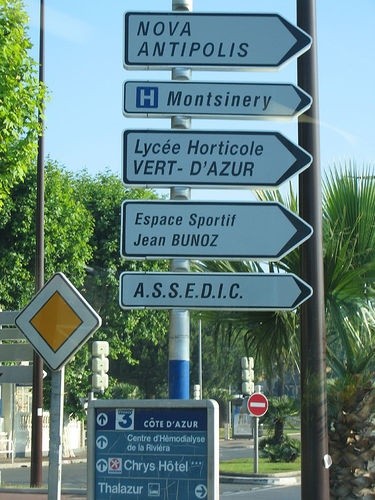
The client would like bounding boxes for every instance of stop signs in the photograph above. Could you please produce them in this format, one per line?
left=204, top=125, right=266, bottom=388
left=247, top=392, right=269, bottom=417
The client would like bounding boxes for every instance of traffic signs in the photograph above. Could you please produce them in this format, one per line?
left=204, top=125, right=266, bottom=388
left=123, top=81, right=314, bottom=120
left=121, top=129, right=313, bottom=187
left=119, top=271, right=313, bottom=311
left=122, top=12, right=313, bottom=71
left=86, top=399, right=219, bottom=500
left=120, top=200, right=313, bottom=261
